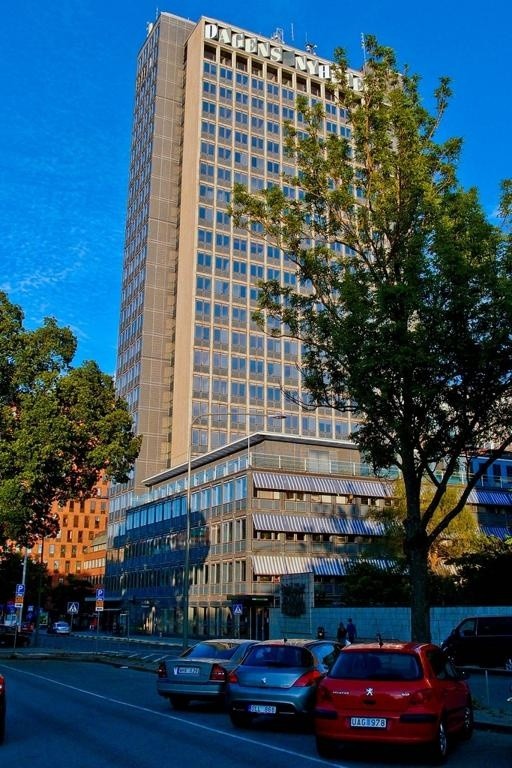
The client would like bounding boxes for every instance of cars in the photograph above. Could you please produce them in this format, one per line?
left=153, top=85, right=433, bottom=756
left=47, top=620, right=70, bottom=635
left=0, top=624, right=30, bottom=649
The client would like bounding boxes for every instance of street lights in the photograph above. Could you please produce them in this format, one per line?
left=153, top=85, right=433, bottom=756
left=181, top=413, right=286, bottom=650
left=19, top=496, right=110, bottom=633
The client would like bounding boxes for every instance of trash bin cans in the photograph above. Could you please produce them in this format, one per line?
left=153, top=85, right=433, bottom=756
left=318, top=627, right=324, bottom=640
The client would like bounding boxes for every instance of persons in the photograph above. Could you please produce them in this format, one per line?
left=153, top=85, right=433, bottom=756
left=347, top=619, right=357, bottom=644
left=337, top=622, right=346, bottom=643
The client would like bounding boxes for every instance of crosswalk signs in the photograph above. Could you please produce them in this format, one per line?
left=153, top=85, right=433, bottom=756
left=67, top=601, right=79, bottom=615
left=233, top=604, right=243, bottom=616
